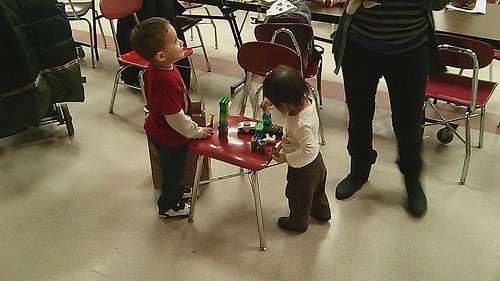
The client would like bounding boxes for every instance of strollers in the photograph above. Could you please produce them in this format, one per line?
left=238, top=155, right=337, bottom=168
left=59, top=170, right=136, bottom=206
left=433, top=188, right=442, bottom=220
left=0, top=0, right=86, bottom=139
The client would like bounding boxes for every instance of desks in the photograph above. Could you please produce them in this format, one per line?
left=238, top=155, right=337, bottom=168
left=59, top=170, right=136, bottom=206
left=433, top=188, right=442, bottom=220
left=182, top=0, right=500, bottom=49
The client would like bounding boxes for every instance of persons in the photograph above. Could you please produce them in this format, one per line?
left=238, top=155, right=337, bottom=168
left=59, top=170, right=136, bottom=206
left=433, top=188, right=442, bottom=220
left=259, top=63, right=332, bottom=234
left=130, top=17, right=212, bottom=218
left=329, top=0, right=447, bottom=217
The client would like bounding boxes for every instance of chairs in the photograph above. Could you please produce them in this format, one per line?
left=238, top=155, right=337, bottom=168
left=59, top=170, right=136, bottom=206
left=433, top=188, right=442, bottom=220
left=65, top=0, right=218, bottom=114
left=188, top=42, right=301, bottom=251
left=425, top=33, right=498, bottom=184
left=254, top=23, right=326, bottom=145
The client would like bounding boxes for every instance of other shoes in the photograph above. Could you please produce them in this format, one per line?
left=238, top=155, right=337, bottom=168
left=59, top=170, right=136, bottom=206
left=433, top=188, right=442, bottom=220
left=335, top=173, right=369, bottom=200
left=182, top=188, right=200, bottom=199
left=405, top=178, right=427, bottom=216
left=278, top=217, right=308, bottom=233
left=159, top=203, right=190, bottom=219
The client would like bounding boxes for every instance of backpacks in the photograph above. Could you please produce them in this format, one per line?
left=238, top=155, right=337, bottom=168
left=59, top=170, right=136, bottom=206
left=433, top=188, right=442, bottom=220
left=264, top=1, right=324, bottom=59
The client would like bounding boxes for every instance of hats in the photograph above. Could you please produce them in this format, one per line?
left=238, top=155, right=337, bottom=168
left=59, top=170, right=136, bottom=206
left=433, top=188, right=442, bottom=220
left=266, top=0, right=297, bottom=17
